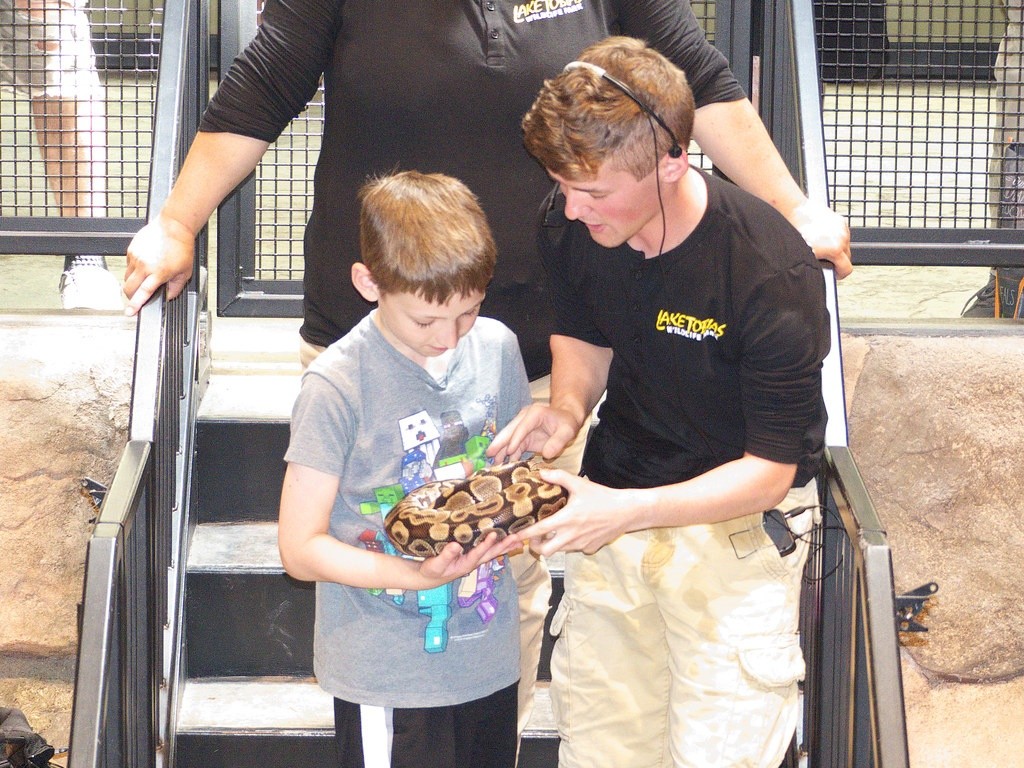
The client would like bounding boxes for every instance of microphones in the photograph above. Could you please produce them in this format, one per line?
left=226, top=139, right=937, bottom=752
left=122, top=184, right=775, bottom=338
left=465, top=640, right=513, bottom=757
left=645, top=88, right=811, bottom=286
left=541, top=183, right=558, bottom=227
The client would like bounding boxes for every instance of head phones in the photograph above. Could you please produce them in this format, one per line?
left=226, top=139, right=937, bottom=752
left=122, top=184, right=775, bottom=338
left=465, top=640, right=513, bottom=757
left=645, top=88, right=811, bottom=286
left=563, top=61, right=682, bottom=158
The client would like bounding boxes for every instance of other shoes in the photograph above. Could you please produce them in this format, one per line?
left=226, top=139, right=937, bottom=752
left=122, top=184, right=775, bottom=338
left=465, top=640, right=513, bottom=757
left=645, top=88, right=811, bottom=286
left=59, top=256, right=125, bottom=311
left=961, top=267, right=995, bottom=317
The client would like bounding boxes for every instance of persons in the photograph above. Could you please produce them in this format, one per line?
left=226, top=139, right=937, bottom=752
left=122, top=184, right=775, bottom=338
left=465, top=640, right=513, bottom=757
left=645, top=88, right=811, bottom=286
left=275, top=174, right=534, bottom=768
left=962, top=0, right=1024, bottom=318
left=123, top=0, right=853, bottom=724
left=0, top=0, right=131, bottom=310
left=487, top=39, right=828, bottom=768
